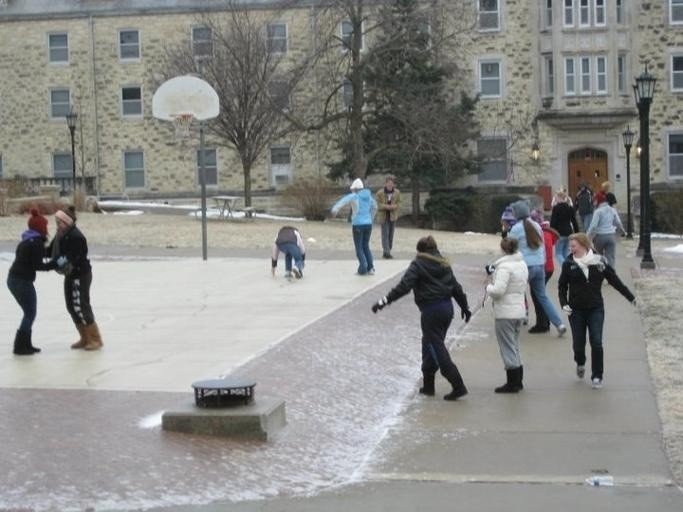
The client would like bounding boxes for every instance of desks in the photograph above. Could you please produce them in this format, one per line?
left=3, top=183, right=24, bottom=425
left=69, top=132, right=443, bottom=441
left=212, top=196, right=240, bottom=220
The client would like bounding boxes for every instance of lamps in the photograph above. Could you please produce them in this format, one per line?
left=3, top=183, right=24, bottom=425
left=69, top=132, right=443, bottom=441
left=532, top=135, right=541, bottom=161
left=636, top=138, right=641, bottom=158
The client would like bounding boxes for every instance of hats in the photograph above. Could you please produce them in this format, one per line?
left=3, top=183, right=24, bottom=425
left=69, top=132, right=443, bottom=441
left=350, top=178, right=364, bottom=189
left=510, top=200, right=529, bottom=220
left=55, top=206, right=76, bottom=226
left=502, top=206, right=516, bottom=225
left=531, top=208, right=542, bottom=224
left=28, top=210, right=48, bottom=235
left=595, top=191, right=607, bottom=203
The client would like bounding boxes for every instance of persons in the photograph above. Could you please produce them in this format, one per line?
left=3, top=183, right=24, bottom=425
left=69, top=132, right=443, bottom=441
left=500, top=205, right=516, bottom=237
left=586, top=191, right=627, bottom=285
left=508, top=200, right=566, bottom=339
left=550, top=191, right=577, bottom=266
left=271, top=226, right=305, bottom=279
left=482, top=236, right=528, bottom=394
left=572, top=181, right=593, bottom=232
left=6, top=209, right=71, bottom=355
left=375, top=175, right=401, bottom=259
left=551, top=185, right=573, bottom=210
left=327, top=177, right=377, bottom=277
left=48, top=206, right=103, bottom=351
left=529, top=209, right=559, bottom=334
left=557, top=233, right=640, bottom=388
left=600, top=181, right=617, bottom=210
left=371, top=236, right=471, bottom=401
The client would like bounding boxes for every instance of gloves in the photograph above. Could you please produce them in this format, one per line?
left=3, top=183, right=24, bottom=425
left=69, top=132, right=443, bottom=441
left=460, top=305, right=472, bottom=322
left=562, top=304, right=572, bottom=315
left=630, top=297, right=639, bottom=308
left=56, top=256, right=68, bottom=267
left=371, top=295, right=390, bottom=314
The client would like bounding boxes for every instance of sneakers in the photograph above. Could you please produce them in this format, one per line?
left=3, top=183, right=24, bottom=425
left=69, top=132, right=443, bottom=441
left=292, top=265, right=302, bottom=278
left=592, top=378, right=602, bottom=389
left=528, top=325, right=549, bottom=333
left=383, top=251, right=392, bottom=258
left=557, top=324, right=566, bottom=336
left=577, top=365, right=585, bottom=377
left=358, top=268, right=376, bottom=275
left=285, top=271, right=291, bottom=277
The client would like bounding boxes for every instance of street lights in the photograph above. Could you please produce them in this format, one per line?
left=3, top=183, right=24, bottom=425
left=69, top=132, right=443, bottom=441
left=621, top=125, right=636, bottom=240
left=63, top=105, right=79, bottom=194
left=632, top=60, right=657, bottom=270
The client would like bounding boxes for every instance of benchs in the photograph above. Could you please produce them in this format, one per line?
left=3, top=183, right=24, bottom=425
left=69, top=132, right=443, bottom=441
left=188, top=207, right=207, bottom=218
left=241, top=207, right=257, bottom=219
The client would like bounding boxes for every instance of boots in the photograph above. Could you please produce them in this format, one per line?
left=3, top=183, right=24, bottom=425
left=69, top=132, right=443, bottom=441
left=495, top=366, right=523, bottom=393
left=12, top=330, right=33, bottom=354
left=418, top=371, right=434, bottom=395
left=441, top=364, right=467, bottom=400
left=85, top=322, right=103, bottom=350
left=27, top=330, right=41, bottom=352
left=71, top=327, right=86, bottom=348
